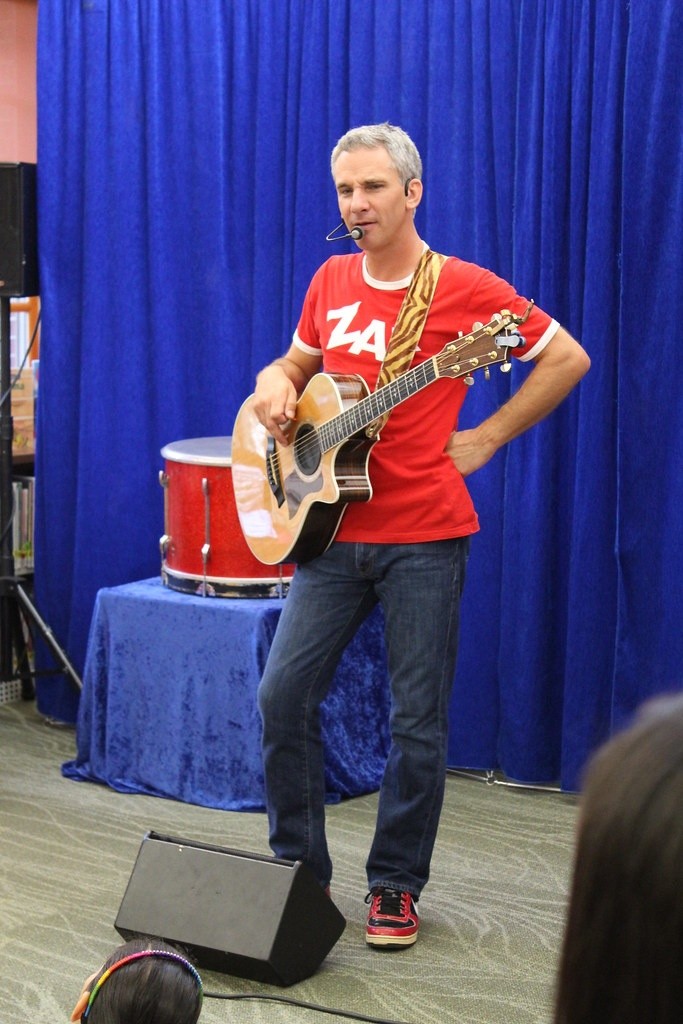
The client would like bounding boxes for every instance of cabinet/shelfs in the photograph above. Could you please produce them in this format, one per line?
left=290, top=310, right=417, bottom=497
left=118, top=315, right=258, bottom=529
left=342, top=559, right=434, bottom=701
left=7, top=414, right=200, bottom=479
left=0, top=160, right=42, bottom=701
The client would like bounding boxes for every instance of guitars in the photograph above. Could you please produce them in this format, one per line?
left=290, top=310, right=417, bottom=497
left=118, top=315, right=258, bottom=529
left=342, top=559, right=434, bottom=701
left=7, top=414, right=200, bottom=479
left=230, top=298, right=535, bottom=566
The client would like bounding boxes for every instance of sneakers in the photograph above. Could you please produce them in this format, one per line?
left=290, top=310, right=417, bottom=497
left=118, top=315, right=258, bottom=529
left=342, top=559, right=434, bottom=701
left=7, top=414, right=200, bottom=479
left=364, top=881, right=421, bottom=953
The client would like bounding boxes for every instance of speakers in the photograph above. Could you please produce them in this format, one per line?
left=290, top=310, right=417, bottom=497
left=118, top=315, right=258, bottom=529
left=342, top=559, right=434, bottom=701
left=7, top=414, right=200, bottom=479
left=0, top=161, right=39, bottom=298
left=112, top=832, right=345, bottom=988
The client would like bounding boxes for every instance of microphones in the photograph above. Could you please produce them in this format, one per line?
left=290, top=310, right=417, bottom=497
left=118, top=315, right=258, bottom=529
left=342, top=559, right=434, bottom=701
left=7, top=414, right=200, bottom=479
left=325, top=222, right=363, bottom=242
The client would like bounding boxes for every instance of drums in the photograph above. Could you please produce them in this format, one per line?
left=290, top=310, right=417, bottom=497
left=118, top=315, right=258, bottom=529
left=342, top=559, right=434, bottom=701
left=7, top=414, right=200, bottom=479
left=158, top=435, right=297, bottom=598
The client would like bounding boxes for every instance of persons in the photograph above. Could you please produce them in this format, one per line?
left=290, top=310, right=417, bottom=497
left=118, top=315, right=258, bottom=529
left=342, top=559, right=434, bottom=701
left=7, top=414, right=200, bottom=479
left=70, top=938, right=204, bottom=1024
left=253, top=122, right=591, bottom=950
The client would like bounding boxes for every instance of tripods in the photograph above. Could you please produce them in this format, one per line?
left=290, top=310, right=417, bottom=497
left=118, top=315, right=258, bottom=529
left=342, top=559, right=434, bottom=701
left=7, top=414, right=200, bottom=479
left=0, top=297, right=84, bottom=701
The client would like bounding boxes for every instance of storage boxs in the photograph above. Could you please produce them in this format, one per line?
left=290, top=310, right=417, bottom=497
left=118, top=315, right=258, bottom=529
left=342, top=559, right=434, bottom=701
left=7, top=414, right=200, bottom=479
left=11, top=367, right=35, bottom=455
